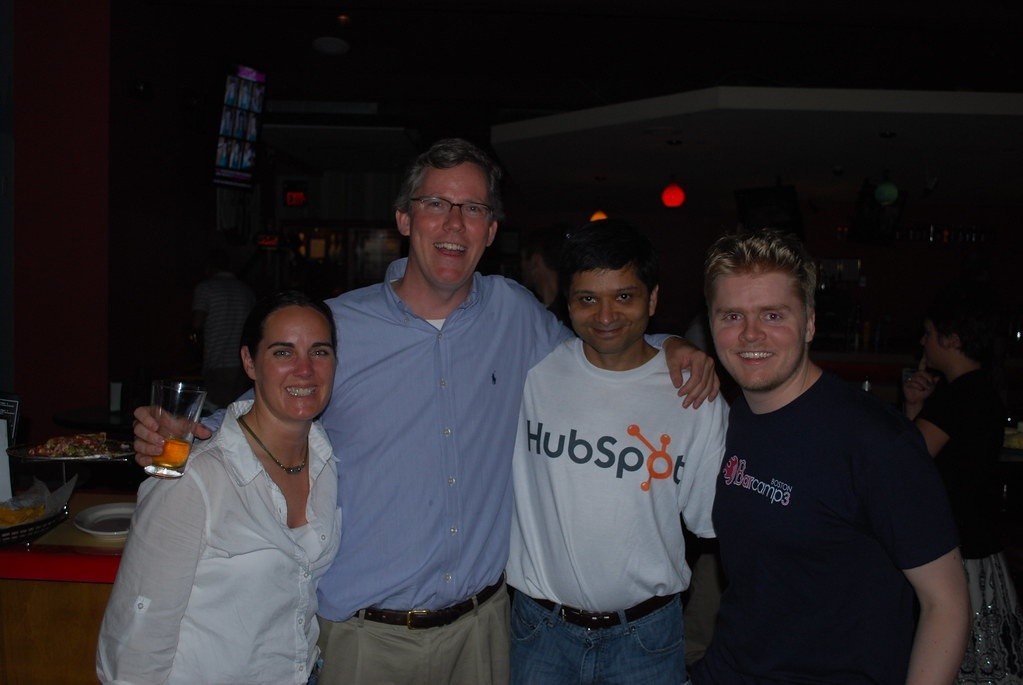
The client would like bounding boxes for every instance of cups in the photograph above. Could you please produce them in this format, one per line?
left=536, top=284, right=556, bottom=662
left=142, top=383, right=207, bottom=480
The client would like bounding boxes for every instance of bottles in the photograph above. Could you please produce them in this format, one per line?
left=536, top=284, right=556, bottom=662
left=814, top=257, right=844, bottom=293
left=892, top=220, right=990, bottom=243
left=832, top=220, right=852, bottom=243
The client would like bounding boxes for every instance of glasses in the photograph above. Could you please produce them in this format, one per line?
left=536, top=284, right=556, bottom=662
left=410, top=195, right=494, bottom=217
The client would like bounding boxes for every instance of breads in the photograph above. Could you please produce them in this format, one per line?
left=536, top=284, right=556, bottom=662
left=0, top=504, right=47, bottom=529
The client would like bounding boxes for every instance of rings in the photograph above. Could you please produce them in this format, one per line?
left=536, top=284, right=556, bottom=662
left=908, top=377, right=911, bottom=381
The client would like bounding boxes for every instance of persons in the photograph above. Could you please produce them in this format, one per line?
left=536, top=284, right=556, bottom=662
left=133, top=138, right=720, bottom=685
left=93, top=292, right=339, bottom=685
left=903, top=304, right=1023, bottom=685
left=692, top=231, right=973, bottom=685
left=521, top=226, right=572, bottom=328
left=191, top=251, right=256, bottom=395
left=686, top=302, right=716, bottom=358
left=507, top=222, right=731, bottom=684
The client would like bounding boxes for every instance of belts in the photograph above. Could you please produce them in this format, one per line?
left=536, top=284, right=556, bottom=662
left=354, top=573, right=504, bottom=630
left=532, top=595, right=675, bottom=630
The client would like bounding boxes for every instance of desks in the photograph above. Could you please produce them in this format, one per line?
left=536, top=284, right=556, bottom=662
left=51, top=407, right=130, bottom=435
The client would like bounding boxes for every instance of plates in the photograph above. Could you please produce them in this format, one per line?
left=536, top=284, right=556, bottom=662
left=71, top=502, right=139, bottom=540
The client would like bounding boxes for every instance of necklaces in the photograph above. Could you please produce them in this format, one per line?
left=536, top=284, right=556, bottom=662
left=239, top=415, right=308, bottom=473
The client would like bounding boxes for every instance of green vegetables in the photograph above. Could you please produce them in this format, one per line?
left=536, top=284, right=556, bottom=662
left=65, top=439, right=133, bottom=456
